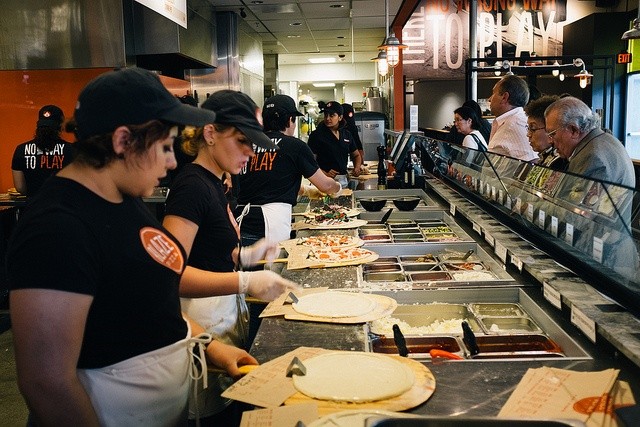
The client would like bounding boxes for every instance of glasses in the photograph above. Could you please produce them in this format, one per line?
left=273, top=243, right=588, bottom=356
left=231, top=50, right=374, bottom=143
left=545, top=127, right=561, bottom=139
left=453, top=118, right=463, bottom=122
left=525, top=125, right=544, bottom=133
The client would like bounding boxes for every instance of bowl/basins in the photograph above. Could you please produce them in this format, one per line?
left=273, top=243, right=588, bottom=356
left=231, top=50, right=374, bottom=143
left=393, top=198, right=420, bottom=211
left=360, top=199, right=387, bottom=212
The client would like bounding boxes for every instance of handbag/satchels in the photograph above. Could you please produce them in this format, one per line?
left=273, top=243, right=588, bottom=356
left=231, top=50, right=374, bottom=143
left=467, top=133, right=488, bottom=172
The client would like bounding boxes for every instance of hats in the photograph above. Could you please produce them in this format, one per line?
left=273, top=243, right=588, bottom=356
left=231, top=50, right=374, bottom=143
left=319, top=101, right=343, bottom=114
left=37, top=105, right=63, bottom=128
left=201, top=90, right=280, bottom=150
left=262, top=94, right=304, bottom=118
left=342, top=104, right=355, bottom=121
left=75, top=67, right=216, bottom=134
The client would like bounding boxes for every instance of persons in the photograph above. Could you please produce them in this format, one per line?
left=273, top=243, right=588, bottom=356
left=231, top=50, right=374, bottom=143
left=232, top=94, right=343, bottom=274
left=521, top=95, right=568, bottom=213
left=544, top=96, right=639, bottom=279
left=6, top=64, right=259, bottom=427
left=306, top=100, right=361, bottom=190
left=481, top=75, right=538, bottom=197
left=340, top=103, right=364, bottom=166
left=449, top=106, right=488, bottom=172
left=446, top=100, right=490, bottom=176
left=156, top=89, right=305, bottom=425
left=11, top=103, right=75, bottom=195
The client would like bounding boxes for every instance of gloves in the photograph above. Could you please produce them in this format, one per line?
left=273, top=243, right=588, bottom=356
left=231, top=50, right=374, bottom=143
left=238, top=270, right=303, bottom=301
left=302, top=185, right=327, bottom=200
left=240, top=238, right=281, bottom=267
left=330, top=182, right=342, bottom=199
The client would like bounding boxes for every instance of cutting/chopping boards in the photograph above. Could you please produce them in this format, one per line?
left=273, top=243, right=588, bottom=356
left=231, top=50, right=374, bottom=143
left=292, top=209, right=361, bottom=219
left=292, top=217, right=368, bottom=230
left=255, top=248, right=380, bottom=267
left=285, top=235, right=367, bottom=249
left=204, top=351, right=437, bottom=409
left=245, top=293, right=397, bottom=323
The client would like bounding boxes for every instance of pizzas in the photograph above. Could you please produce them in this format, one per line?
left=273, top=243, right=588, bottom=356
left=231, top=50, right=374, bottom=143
left=297, top=236, right=357, bottom=249
left=9, top=187, right=20, bottom=194
left=292, top=351, right=416, bottom=403
left=291, top=291, right=375, bottom=318
left=308, top=249, right=372, bottom=262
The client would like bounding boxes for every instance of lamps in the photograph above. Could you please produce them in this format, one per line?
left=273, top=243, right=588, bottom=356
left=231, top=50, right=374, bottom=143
left=376, top=33, right=407, bottom=70
left=370, top=51, right=388, bottom=77
left=558, top=71, right=566, bottom=82
left=550, top=58, right=559, bottom=78
left=621, top=3, right=640, bottom=39
left=572, top=56, right=594, bottom=90
